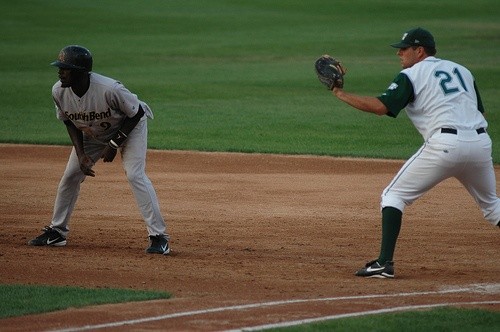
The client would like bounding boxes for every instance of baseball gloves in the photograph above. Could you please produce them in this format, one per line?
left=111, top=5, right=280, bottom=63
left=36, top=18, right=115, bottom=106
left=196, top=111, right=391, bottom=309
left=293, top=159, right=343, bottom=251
left=314, top=53, right=347, bottom=92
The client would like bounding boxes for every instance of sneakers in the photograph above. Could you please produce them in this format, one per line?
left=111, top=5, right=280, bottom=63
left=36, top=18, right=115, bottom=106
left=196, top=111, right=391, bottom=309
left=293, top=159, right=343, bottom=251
left=145, top=235, right=171, bottom=255
left=354, top=258, right=395, bottom=279
left=25, top=226, right=66, bottom=247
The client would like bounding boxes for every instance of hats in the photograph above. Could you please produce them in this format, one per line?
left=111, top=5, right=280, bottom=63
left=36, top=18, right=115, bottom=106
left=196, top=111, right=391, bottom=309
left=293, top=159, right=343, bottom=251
left=390, top=28, right=438, bottom=55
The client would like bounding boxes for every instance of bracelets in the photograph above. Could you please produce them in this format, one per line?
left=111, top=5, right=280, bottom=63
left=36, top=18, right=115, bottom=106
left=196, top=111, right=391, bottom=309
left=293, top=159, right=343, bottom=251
left=109, top=142, right=118, bottom=150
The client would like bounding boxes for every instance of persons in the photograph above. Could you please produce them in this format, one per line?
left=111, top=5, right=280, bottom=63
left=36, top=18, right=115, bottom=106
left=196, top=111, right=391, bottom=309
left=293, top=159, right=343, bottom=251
left=315, top=28, right=500, bottom=280
left=28, top=45, right=170, bottom=254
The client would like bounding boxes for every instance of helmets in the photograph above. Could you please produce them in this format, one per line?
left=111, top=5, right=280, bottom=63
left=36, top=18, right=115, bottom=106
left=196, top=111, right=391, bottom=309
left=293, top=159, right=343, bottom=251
left=49, top=45, right=93, bottom=71
